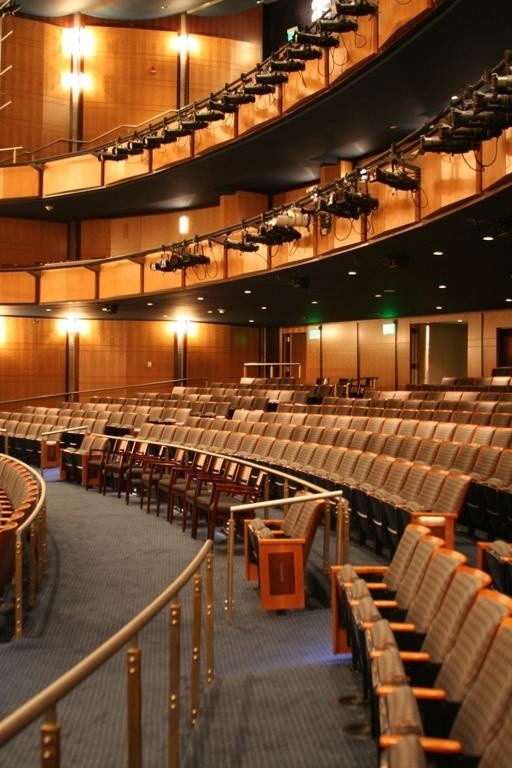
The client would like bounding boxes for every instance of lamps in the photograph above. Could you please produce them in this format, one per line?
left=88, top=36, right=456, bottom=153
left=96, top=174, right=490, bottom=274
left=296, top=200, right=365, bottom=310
left=383, top=319, right=399, bottom=334
left=309, top=325, right=323, bottom=339
